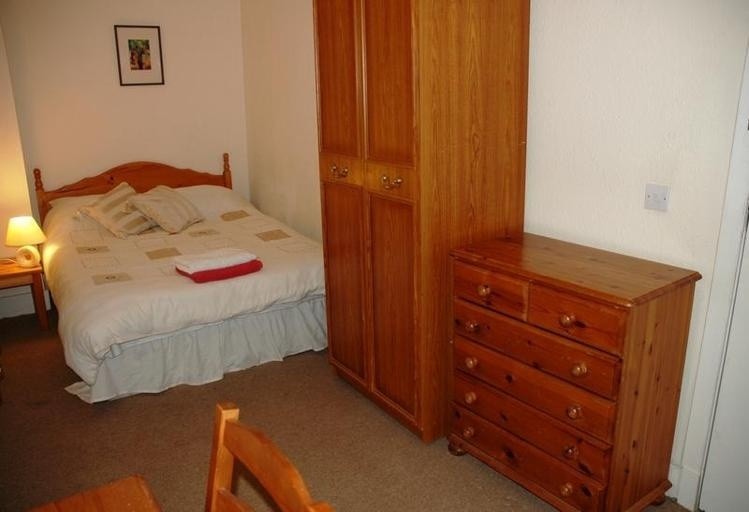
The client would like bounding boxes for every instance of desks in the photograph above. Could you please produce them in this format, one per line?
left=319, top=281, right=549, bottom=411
left=24, top=474, right=163, bottom=512
left=0, top=257, right=50, bottom=331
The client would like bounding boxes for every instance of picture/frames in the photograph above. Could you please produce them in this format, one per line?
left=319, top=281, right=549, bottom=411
left=114, top=25, right=164, bottom=86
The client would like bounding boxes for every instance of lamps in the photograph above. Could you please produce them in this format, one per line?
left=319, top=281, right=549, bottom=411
left=5, top=216, right=47, bottom=259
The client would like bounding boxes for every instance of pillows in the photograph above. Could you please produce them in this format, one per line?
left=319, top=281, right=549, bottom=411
left=127, top=184, right=205, bottom=234
left=80, top=181, right=160, bottom=240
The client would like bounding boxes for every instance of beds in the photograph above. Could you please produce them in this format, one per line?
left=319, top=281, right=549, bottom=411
left=34, top=153, right=329, bottom=404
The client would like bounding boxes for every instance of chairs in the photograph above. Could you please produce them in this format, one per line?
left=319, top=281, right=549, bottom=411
left=204, top=400, right=335, bottom=512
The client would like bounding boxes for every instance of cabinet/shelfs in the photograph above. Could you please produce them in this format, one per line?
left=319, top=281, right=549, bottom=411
left=312, top=0, right=530, bottom=445
left=448, top=232, right=703, bottom=512
